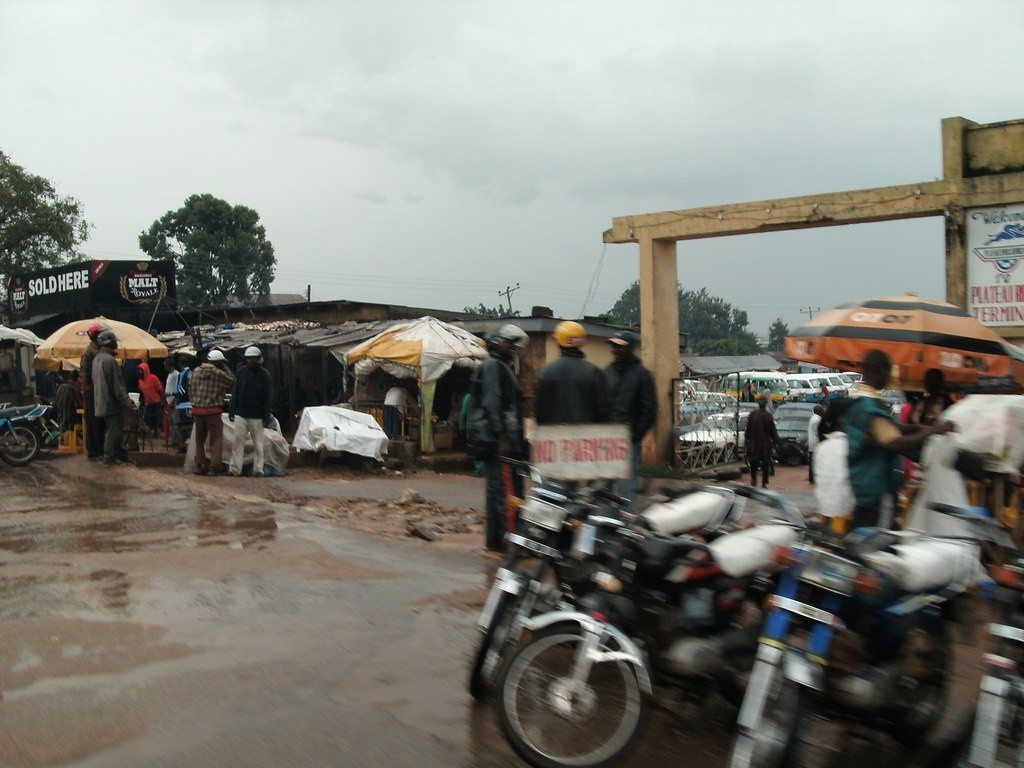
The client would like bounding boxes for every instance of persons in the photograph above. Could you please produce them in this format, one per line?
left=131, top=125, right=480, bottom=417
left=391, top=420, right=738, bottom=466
left=808, top=349, right=958, bottom=531
left=462, top=322, right=529, bottom=555
left=383, top=383, right=419, bottom=441
left=744, top=398, right=780, bottom=487
left=189, top=350, right=237, bottom=477
left=742, top=379, right=830, bottom=410
left=604, top=332, right=659, bottom=507
left=45, top=370, right=81, bottom=389
left=530, top=321, right=610, bottom=490
left=81, top=325, right=136, bottom=467
left=226, top=346, right=273, bottom=478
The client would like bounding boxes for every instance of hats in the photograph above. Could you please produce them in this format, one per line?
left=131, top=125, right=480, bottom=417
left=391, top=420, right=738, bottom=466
left=606, top=331, right=635, bottom=347
left=207, top=350, right=229, bottom=362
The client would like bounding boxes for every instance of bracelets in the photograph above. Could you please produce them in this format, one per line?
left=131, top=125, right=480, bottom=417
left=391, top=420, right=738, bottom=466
left=170, top=391, right=174, bottom=396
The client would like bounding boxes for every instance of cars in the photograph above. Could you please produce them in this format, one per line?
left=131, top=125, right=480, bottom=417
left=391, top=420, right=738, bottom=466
left=882, top=389, right=906, bottom=422
left=678, top=380, right=825, bottom=465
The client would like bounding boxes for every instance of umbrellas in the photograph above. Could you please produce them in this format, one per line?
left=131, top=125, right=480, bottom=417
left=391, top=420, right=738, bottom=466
left=138, top=354, right=195, bottom=453
left=163, top=401, right=172, bottom=452
left=347, top=316, right=490, bottom=448
left=33, top=319, right=167, bottom=373
left=784, top=292, right=1024, bottom=396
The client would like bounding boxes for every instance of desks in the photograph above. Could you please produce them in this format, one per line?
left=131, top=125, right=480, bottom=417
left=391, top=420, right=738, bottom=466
left=406, top=422, right=456, bottom=451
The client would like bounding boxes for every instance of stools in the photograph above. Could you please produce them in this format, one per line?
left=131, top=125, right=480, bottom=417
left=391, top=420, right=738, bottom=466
left=58, top=424, right=82, bottom=453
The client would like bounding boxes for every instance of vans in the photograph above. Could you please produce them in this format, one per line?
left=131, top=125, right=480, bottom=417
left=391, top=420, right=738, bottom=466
left=718, top=372, right=862, bottom=409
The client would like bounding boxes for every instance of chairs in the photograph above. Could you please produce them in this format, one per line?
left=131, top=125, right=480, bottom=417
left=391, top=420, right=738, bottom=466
left=123, top=424, right=154, bottom=452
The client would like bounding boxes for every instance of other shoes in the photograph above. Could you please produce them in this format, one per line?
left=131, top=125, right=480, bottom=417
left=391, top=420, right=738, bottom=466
left=104, top=457, right=122, bottom=467
left=88, top=452, right=102, bottom=460
left=762, top=484, right=768, bottom=489
left=225, top=469, right=238, bottom=477
left=751, top=480, right=756, bottom=486
left=255, top=473, right=264, bottom=478
left=192, top=466, right=219, bottom=477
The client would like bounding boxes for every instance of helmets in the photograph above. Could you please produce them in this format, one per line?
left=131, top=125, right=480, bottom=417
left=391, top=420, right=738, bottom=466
left=98, top=332, right=120, bottom=345
left=486, top=325, right=522, bottom=353
left=245, top=347, right=262, bottom=359
left=553, top=321, right=587, bottom=348
left=88, top=324, right=104, bottom=338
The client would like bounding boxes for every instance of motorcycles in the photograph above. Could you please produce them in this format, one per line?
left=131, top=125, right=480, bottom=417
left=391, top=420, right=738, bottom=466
left=470, top=453, right=1023, bottom=768
left=0, top=402, right=61, bottom=467
